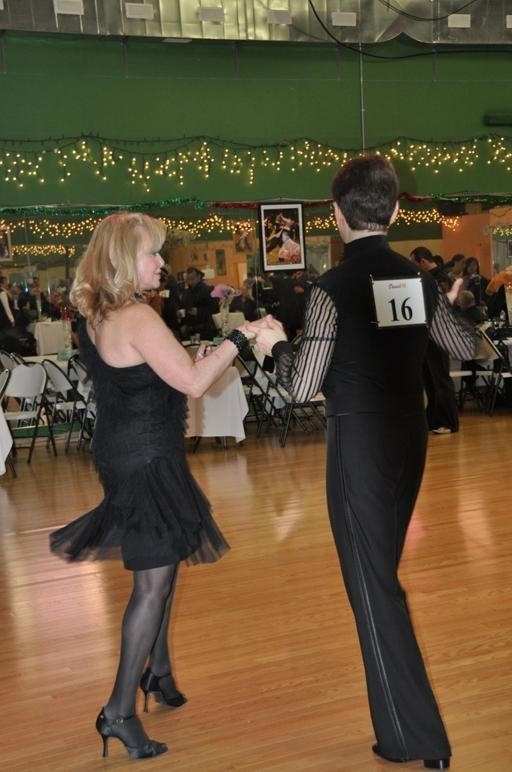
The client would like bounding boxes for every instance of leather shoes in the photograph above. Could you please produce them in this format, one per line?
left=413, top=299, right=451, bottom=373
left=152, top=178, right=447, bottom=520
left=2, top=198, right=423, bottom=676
left=372, top=742, right=450, bottom=769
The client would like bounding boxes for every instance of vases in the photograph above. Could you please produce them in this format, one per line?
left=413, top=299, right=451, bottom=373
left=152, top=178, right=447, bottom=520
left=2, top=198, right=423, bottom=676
left=222, top=315, right=230, bottom=337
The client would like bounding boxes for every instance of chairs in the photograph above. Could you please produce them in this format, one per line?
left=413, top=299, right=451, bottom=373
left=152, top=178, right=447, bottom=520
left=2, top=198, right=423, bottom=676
left=0, top=328, right=512, bottom=479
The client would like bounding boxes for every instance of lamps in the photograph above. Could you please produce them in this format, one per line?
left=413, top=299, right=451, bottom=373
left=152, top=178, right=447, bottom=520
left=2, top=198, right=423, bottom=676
left=258, top=203, right=307, bottom=272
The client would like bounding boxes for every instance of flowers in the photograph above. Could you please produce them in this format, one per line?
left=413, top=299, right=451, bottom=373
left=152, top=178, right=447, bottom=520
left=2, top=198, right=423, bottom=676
left=209, top=284, right=241, bottom=322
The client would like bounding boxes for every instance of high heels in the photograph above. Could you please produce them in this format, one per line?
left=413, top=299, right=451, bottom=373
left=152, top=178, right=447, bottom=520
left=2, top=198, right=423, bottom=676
left=140, top=667, right=187, bottom=713
left=96, top=706, right=168, bottom=759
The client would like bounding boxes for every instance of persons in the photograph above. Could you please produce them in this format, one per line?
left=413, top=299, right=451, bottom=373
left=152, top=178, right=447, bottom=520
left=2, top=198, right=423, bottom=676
left=144, top=268, right=318, bottom=349
left=265, top=213, right=301, bottom=263
left=255, top=155, right=451, bottom=768
left=433, top=253, right=511, bottom=372
left=50, top=210, right=284, bottom=760
left=410, top=246, right=459, bottom=434
left=1, top=273, right=80, bottom=351
